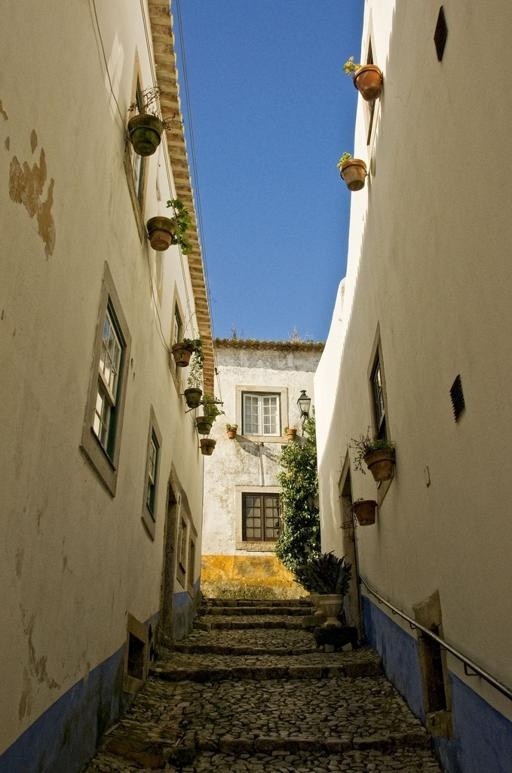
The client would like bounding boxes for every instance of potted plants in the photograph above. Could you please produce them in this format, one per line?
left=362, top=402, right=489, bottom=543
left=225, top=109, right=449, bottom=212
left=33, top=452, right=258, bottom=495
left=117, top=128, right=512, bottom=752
left=346, top=424, right=397, bottom=481
left=146, top=197, right=195, bottom=255
left=343, top=53, right=384, bottom=100
left=338, top=494, right=379, bottom=536
left=226, top=421, right=240, bottom=440
left=125, top=76, right=180, bottom=156
left=337, top=150, right=370, bottom=190
left=171, top=330, right=221, bottom=457
left=283, top=425, right=298, bottom=441
left=294, top=548, right=354, bottom=636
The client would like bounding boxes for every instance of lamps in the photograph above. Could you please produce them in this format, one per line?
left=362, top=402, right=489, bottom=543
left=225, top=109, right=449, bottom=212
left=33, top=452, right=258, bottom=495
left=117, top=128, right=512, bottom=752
left=296, top=389, right=313, bottom=420
